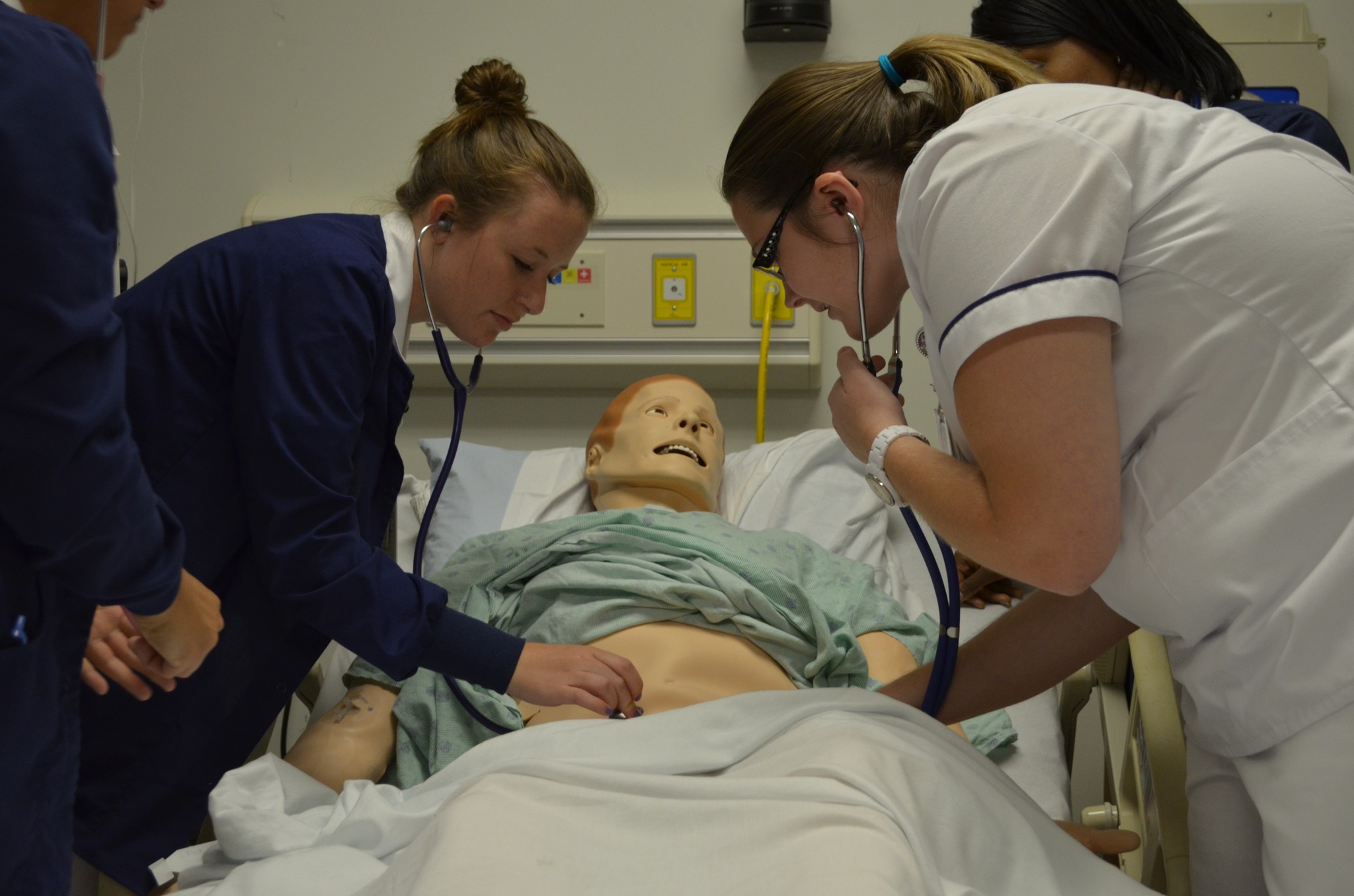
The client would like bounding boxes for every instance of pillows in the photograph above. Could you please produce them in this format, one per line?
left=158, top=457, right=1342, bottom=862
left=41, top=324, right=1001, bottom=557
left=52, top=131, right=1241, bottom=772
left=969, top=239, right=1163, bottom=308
left=415, top=429, right=907, bottom=606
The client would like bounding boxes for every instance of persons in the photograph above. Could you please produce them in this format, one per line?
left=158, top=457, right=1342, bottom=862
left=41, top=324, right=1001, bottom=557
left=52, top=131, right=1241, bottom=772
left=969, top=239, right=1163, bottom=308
left=60, top=55, right=600, bottom=896
left=950, top=0, right=1352, bottom=614
left=0, top=0, right=227, bottom=896
left=284, top=371, right=1019, bottom=797
left=717, top=34, right=1354, bottom=896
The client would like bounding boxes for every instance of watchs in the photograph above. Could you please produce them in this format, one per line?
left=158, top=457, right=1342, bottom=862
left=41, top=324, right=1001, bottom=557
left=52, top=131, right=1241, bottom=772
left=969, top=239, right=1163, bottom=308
left=864, top=424, right=931, bottom=509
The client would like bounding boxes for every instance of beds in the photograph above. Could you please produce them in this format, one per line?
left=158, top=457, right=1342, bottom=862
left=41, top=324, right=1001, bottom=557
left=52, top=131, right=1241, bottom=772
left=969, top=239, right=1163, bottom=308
left=237, top=473, right=1192, bottom=895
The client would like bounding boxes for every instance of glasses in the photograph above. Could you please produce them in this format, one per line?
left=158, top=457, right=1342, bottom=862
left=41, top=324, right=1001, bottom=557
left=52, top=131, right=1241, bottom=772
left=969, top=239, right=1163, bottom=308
left=752, top=174, right=859, bottom=281
